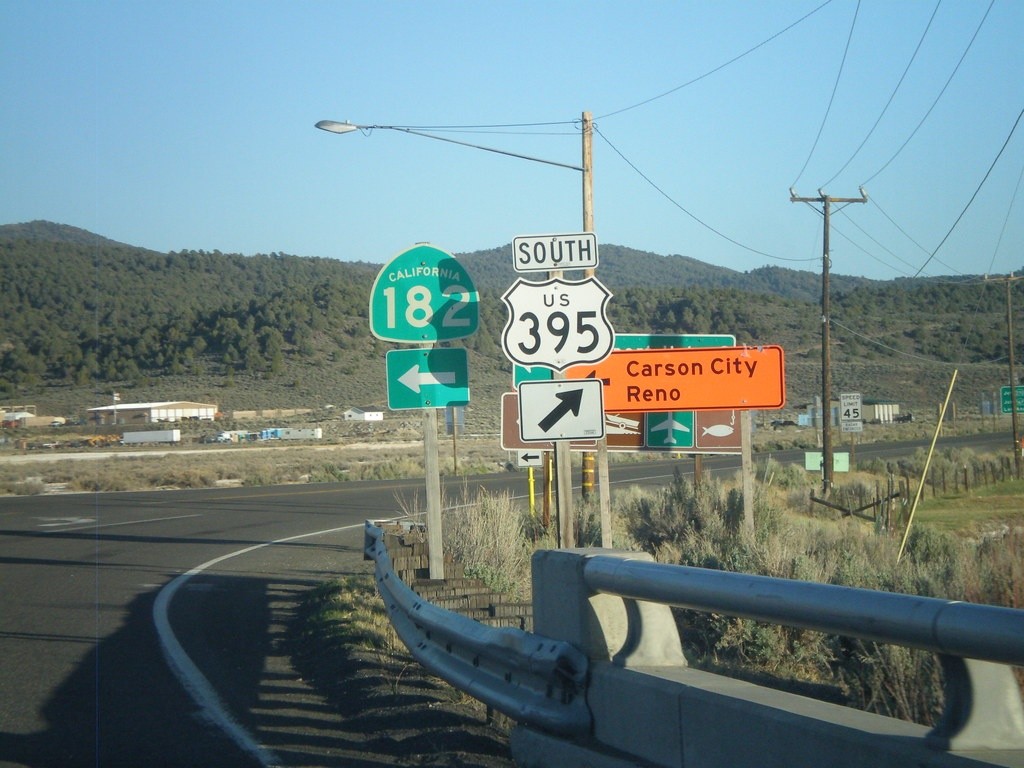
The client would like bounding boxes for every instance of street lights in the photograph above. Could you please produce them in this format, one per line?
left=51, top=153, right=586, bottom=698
left=314, top=112, right=602, bottom=502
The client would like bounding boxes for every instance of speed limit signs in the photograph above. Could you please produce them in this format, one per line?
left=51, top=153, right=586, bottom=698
left=840, top=394, right=862, bottom=422
